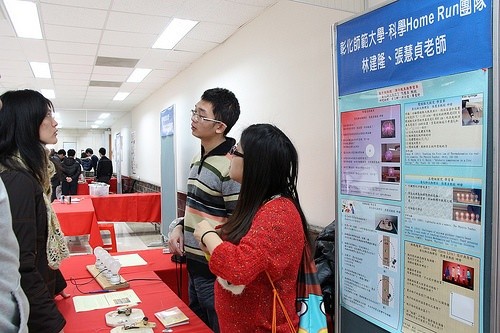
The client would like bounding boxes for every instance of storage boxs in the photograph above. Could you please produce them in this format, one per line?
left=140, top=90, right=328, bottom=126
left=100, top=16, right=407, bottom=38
left=88, top=184, right=110, bottom=196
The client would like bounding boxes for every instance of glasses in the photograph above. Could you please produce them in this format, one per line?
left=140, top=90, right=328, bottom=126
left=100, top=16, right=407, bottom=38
left=229, top=145, right=244, bottom=158
left=45, top=112, right=55, bottom=119
left=191, top=110, right=227, bottom=127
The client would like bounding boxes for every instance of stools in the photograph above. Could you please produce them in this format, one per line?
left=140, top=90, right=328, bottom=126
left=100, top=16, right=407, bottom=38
left=93, top=223, right=117, bottom=253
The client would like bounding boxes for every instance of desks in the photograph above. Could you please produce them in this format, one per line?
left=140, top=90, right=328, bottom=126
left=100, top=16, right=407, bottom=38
left=59, top=248, right=189, bottom=304
left=54, top=192, right=161, bottom=232
left=51, top=198, right=103, bottom=249
left=54, top=271, right=214, bottom=333
left=77, top=178, right=117, bottom=195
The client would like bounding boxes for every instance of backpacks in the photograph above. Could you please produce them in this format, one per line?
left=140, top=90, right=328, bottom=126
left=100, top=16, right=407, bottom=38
left=260, top=191, right=328, bottom=333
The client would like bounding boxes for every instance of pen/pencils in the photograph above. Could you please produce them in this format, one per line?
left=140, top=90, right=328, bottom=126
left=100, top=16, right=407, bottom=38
left=83, top=288, right=117, bottom=293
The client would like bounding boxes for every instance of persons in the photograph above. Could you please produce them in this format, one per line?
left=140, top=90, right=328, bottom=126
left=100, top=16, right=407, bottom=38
left=79, top=153, right=92, bottom=176
left=0, top=90, right=70, bottom=333
left=193, top=124, right=322, bottom=332
left=86, top=148, right=99, bottom=175
left=169, top=87, right=240, bottom=332
left=50, top=149, right=66, bottom=202
left=0, top=179, right=30, bottom=332
left=96, top=148, right=113, bottom=192
left=58, top=149, right=81, bottom=195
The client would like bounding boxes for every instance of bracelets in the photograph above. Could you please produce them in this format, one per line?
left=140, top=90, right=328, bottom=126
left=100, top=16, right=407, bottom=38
left=200, top=231, right=219, bottom=246
